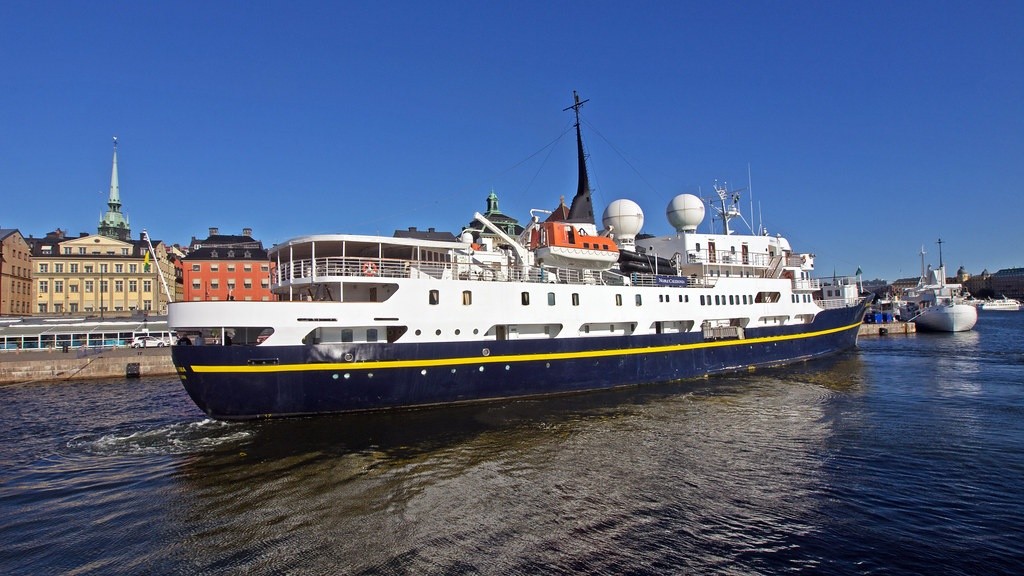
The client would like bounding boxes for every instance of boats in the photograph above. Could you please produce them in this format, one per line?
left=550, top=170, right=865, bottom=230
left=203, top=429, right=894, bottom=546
left=982, top=298, right=1019, bottom=311
left=899, top=237, right=978, bottom=334
left=165, top=89, right=875, bottom=423
left=535, top=221, right=619, bottom=271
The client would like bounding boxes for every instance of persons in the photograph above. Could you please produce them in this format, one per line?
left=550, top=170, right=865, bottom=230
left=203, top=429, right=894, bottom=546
left=194, top=331, right=206, bottom=346
left=176, top=333, right=192, bottom=346
left=221, top=332, right=233, bottom=345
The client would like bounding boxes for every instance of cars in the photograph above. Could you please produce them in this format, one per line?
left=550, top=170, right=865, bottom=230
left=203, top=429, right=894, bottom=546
left=131, top=336, right=170, bottom=348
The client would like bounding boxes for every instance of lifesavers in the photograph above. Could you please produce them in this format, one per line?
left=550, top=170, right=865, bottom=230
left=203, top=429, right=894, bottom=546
left=363, top=261, right=378, bottom=277
left=785, top=272, right=792, bottom=278
